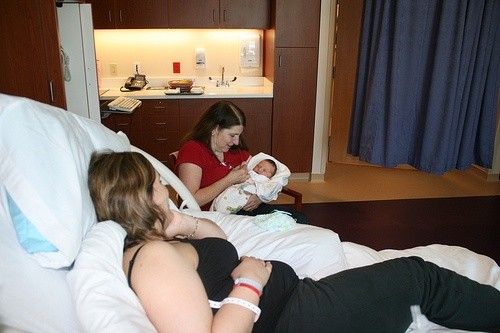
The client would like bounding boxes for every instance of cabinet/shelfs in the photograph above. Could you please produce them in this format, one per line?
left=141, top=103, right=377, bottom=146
left=0, top=0, right=321, bottom=178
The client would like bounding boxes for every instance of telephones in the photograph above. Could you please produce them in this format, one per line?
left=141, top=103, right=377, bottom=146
left=124, top=74, right=149, bottom=91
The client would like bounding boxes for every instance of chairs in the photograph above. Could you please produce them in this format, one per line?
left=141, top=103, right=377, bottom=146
left=167, top=150, right=309, bottom=226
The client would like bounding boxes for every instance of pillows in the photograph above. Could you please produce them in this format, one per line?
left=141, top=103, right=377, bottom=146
left=0, top=93, right=130, bottom=270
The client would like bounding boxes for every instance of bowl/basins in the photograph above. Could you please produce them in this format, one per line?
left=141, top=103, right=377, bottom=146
left=168, top=79, right=193, bottom=92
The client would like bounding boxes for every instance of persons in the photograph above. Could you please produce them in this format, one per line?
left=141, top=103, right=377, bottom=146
left=209, top=152, right=291, bottom=214
left=88, top=152, right=500, bottom=333
left=177, top=102, right=308, bottom=224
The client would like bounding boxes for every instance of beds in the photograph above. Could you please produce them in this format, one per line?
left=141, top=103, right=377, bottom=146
left=0, top=93, right=500, bottom=333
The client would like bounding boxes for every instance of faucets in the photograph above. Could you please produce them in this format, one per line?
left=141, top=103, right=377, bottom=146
left=209, top=64, right=237, bottom=87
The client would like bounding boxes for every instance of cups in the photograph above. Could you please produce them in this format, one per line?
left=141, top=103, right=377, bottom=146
left=173, top=62, right=180, bottom=73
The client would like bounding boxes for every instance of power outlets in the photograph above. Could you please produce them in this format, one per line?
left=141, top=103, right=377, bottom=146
left=110, top=63, right=119, bottom=77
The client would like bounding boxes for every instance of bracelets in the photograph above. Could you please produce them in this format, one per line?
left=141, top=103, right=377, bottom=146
left=234, top=278, right=263, bottom=297
left=184, top=216, right=198, bottom=239
left=209, top=297, right=261, bottom=322
